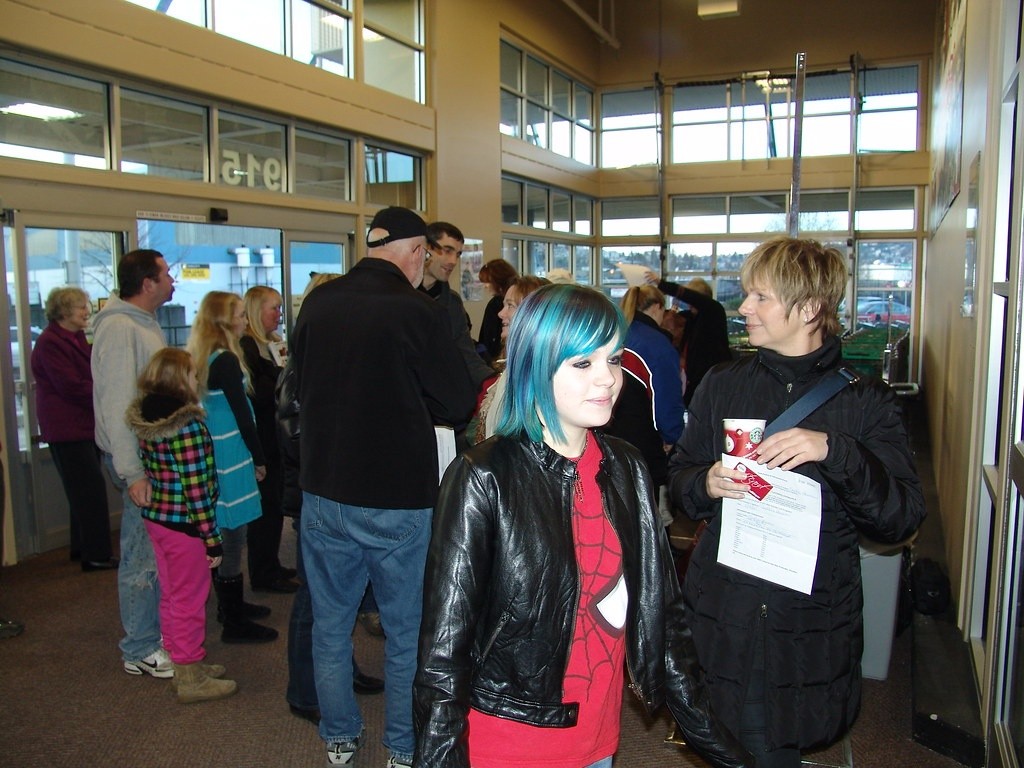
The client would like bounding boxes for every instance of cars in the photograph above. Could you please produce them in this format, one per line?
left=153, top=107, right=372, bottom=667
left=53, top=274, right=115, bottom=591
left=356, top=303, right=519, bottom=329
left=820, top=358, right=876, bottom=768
left=839, top=295, right=911, bottom=331
left=8, top=326, right=48, bottom=369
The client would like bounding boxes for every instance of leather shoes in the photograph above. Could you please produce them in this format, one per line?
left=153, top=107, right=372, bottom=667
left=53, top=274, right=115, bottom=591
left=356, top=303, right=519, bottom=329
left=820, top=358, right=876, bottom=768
left=72, top=552, right=86, bottom=561
left=82, top=556, right=120, bottom=571
left=352, top=674, right=385, bottom=694
left=289, top=704, right=321, bottom=725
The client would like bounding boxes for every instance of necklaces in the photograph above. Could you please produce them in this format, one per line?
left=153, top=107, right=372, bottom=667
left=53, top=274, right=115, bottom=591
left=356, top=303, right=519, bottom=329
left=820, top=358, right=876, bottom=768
left=562, top=437, right=589, bottom=504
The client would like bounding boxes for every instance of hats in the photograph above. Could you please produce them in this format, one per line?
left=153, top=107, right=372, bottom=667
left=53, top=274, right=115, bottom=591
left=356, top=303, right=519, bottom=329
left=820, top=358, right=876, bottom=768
left=366, top=206, right=442, bottom=249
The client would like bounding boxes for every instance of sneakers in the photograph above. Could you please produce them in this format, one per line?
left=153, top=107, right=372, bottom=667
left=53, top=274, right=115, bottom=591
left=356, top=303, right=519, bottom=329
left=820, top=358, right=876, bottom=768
left=326, top=730, right=366, bottom=768
left=386, top=756, right=413, bottom=768
left=123, top=634, right=173, bottom=678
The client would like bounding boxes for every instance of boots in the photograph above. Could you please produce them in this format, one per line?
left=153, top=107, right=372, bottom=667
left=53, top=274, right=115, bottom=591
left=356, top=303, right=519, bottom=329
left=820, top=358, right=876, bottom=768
left=215, top=573, right=270, bottom=623
left=173, top=661, right=238, bottom=703
left=174, top=661, right=226, bottom=683
left=218, top=575, right=279, bottom=642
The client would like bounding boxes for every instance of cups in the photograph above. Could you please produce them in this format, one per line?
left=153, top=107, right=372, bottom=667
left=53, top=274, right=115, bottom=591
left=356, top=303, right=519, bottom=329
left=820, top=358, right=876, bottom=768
left=722, top=418, right=767, bottom=463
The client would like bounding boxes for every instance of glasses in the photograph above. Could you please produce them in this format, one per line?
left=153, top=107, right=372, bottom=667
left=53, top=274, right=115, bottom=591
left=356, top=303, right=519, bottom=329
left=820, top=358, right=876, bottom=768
left=413, top=245, right=432, bottom=261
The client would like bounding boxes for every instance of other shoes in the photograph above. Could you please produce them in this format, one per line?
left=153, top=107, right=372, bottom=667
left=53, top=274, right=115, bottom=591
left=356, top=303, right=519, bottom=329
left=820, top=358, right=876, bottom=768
left=0, top=618, right=24, bottom=639
left=251, top=575, right=299, bottom=592
left=358, top=612, right=384, bottom=635
left=279, top=567, right=296, bottom=578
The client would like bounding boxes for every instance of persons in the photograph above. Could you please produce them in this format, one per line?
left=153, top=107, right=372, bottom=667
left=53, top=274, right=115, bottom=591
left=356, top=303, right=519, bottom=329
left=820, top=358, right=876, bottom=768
left=91, top=247, right=175, bottom=679
left=185, top=291, right=280, bottom=645
left=31, top=288, right=119, bottom=573
left=125, top=349, right=238, bottom=704
left=621, top=241, right=930, bottom=768
left=239, top=285, right=301, bottom=592
left=288, top=203, right=669, bottom=768
